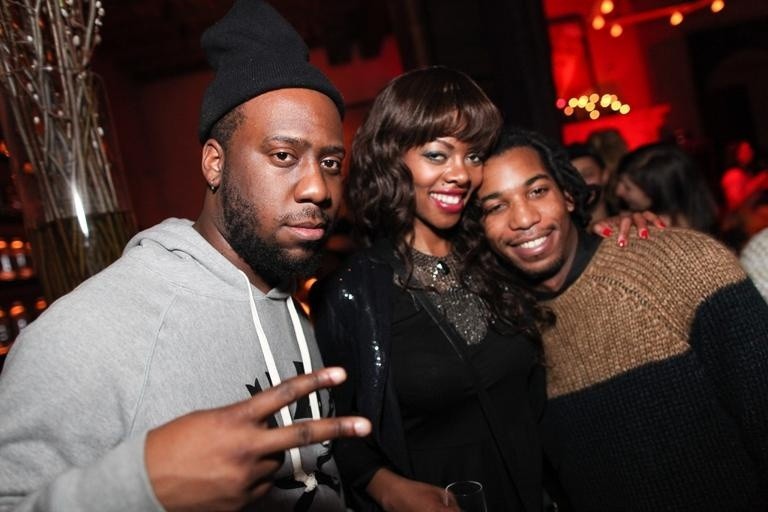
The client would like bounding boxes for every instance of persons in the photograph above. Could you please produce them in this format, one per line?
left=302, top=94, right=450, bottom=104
left=0, top=2, right=372, bottom=512
left=305, top=64, right=666, bottom=511
left=451, top=127, right=767, bottom=512
left=565, top=121, right=768, bottom=300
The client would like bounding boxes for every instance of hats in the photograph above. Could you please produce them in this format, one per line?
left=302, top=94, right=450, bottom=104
left=197, top=7, right=345, bottom=140
